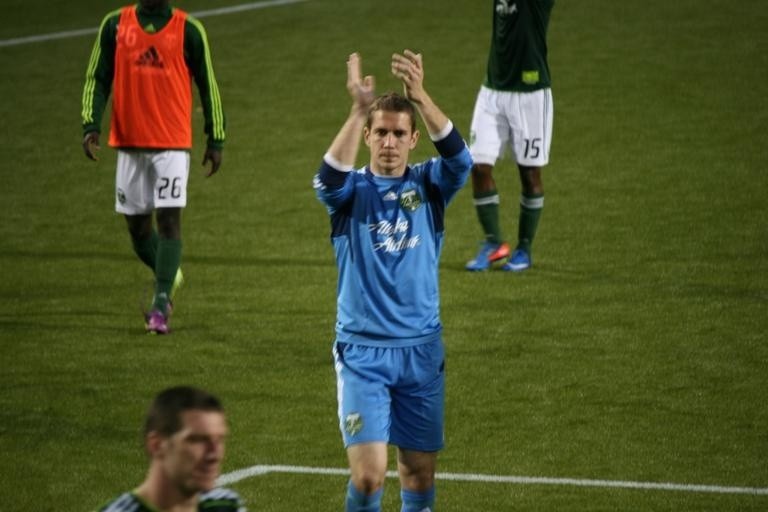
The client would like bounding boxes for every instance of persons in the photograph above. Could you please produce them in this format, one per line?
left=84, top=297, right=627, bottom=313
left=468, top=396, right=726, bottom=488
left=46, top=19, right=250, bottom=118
left=90, top=384, right=248, bottom=512
left=312, top=48, right=474, bottom=512
left=464, top=0, right=556, bottom=272
left=81, top=0, right=226, bottom=335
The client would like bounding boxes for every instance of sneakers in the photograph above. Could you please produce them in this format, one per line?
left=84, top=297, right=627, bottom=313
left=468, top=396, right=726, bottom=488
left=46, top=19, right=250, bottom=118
left=146, top=310, right=168, bottom=334
left=500, top=249, right=531, bottom=271
left=464, top=241, right=510, bottom=271
left=150, top=267, right=183, bottom=312
